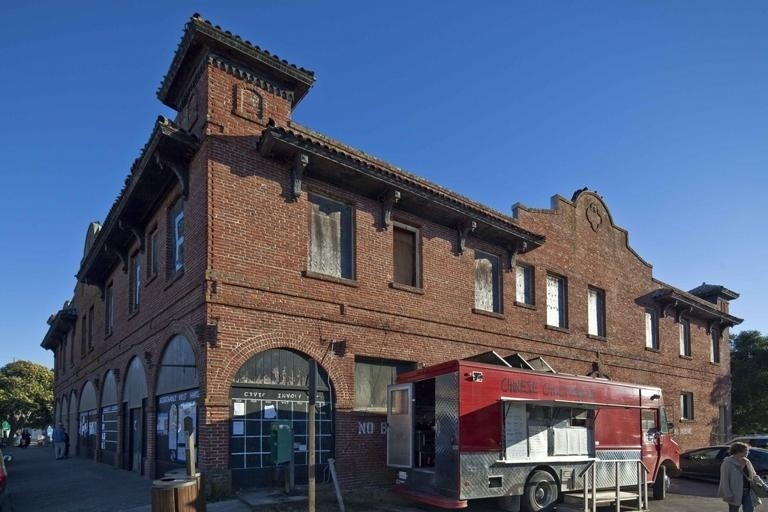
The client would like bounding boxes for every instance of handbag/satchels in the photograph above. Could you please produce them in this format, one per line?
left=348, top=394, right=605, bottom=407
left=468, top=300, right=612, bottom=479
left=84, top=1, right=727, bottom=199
left=750, top=475, right=768, bottom=507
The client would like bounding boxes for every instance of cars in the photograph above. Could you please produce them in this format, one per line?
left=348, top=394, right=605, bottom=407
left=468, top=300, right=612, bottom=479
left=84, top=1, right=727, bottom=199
left=680, top=433, right=768, bottom=486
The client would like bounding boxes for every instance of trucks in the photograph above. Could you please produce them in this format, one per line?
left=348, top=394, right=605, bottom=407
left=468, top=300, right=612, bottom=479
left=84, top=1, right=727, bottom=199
left=386, top=359, right=680, bottom=512
left=169, top=401, right=198, bottom=463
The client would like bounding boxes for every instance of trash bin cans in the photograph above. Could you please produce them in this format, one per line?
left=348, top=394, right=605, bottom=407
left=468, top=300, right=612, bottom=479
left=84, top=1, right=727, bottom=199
left=151, top=477, right=197, bottom=512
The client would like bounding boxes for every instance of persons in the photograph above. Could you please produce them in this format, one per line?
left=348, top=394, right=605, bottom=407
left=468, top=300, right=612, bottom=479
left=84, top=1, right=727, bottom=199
left=21, top=430, right=31, bottom=448
left=62, top=428, right=70, bottom=458
left=53, top=423, right=67, bottom=459
left=718, top=442, right=762, bottom=512
